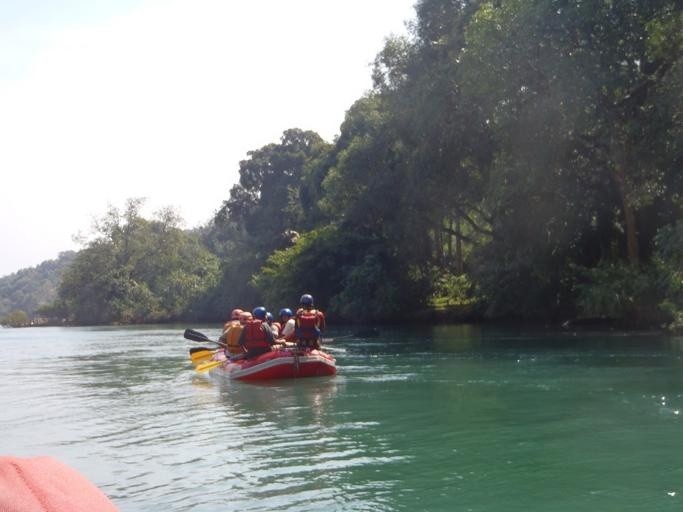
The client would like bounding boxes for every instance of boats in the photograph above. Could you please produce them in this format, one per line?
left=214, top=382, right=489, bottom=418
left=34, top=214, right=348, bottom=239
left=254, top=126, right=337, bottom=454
left=223, top=377, right=339, bottom=421
left=0, top=458, right=114, bottom=512
left=206, top=345, right=342, bottom=381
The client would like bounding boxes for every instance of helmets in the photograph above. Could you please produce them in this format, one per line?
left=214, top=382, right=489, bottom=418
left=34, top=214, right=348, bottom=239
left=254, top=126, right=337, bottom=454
left=266, top=312, right=273, bottom=320
left=239, top=312, right=252, bottom=322
left=253, top=307, right=266, bottom=319
left=299, top=294, right=314, bottom=306
left=231, top=308, right=243, bottom=319
left=279, top=307, right=293, bottom=317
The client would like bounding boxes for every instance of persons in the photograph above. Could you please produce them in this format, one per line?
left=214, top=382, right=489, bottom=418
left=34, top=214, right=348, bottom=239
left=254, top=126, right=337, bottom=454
left=279, top=309, right=295, bottom=342
left=267, top=312, right=279, bottom=339
left=218, top=312, right=252, bottom=360
left=223, top=308, right=242, bottom=333
left=295, top=294, right=326, bottom=348
left=239, top=307, right=285, bottom=358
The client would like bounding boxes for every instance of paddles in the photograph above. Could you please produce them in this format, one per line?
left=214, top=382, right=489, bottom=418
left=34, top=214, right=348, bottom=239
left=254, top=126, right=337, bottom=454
left=196, top=353, right=244, bottom=373
left=190, top=346, right=224, bottom=354
left=184, top=329, right=227, bottom=348
left=192, top=350, right=225, bottom=363
